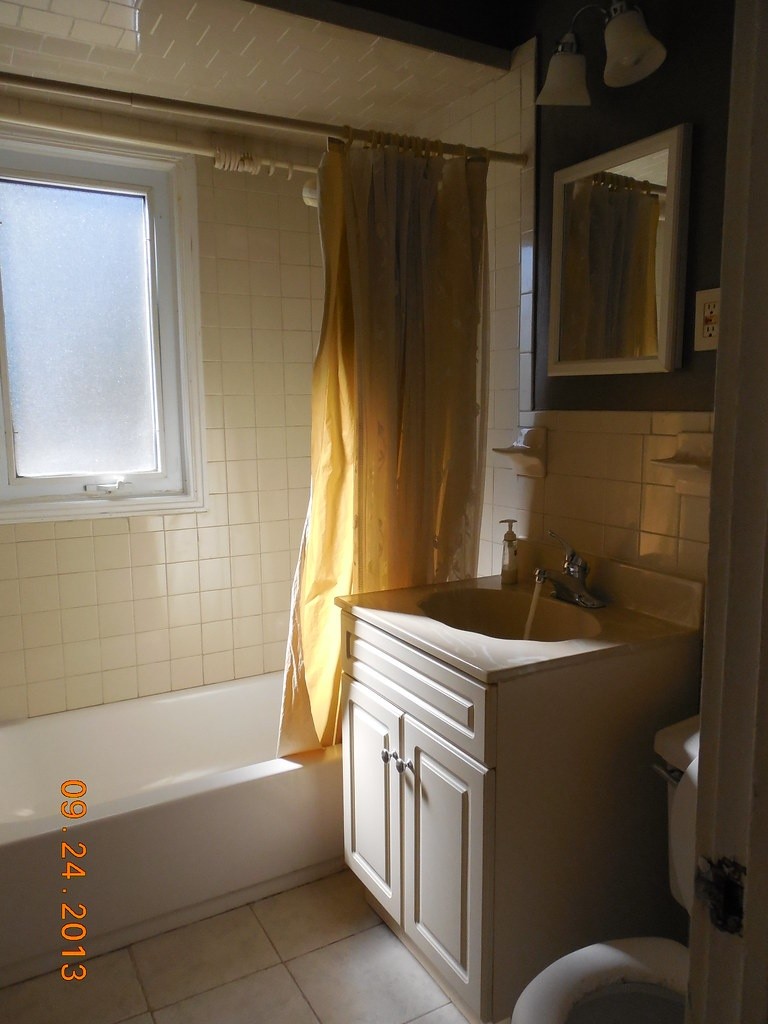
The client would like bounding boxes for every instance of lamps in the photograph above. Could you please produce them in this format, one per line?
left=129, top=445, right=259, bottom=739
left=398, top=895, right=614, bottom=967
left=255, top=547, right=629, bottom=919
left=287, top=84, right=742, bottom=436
left=536, top=0, right=666, bottom=106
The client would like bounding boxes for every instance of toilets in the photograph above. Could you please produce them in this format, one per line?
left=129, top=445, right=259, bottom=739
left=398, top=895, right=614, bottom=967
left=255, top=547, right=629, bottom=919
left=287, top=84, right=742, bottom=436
left=511, top=714, right=701, bottom=1023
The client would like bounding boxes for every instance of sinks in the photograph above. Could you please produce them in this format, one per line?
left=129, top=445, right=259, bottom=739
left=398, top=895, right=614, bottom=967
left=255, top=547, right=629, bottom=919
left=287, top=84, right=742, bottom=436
left=418, top=588, right=599, bottom=642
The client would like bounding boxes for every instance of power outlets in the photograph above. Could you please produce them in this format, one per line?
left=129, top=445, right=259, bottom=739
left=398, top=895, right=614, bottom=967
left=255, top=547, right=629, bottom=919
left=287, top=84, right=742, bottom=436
left=695, top=288, right=720, bottom=351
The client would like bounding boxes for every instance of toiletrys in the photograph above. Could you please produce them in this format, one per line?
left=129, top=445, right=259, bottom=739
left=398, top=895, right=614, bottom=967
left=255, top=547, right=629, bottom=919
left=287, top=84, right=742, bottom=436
left=499, top=519, right=519, bottom=585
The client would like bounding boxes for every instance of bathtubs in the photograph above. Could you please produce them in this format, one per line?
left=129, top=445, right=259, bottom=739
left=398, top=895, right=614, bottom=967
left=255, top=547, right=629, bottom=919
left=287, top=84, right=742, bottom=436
left=1, top=668, right=340, bottom=990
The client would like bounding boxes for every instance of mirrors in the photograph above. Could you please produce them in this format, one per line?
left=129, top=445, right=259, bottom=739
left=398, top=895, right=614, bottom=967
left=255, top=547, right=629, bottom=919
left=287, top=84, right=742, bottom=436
left=546, top=121, right=683, bottom=378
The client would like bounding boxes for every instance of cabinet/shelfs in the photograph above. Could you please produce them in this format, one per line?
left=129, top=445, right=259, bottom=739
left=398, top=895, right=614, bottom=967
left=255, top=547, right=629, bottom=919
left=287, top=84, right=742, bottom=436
left=336, top=610, right=496, bottom=1024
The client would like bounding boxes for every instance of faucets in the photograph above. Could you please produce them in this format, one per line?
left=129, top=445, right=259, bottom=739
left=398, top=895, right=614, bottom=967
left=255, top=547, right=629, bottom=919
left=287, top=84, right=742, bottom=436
left=533, top=528, right=606, bottom=609
